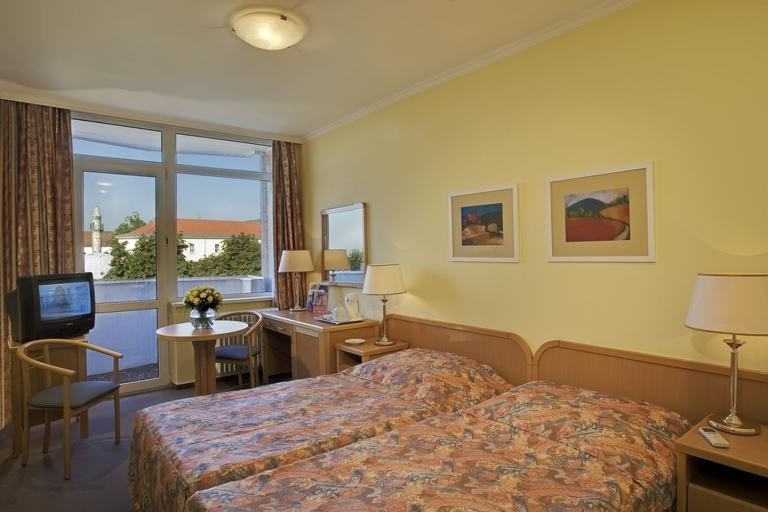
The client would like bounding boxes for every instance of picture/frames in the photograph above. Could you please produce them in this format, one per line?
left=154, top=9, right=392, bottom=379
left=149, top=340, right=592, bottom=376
left=544, top=161, right=658, bottom=264
left=446, top=184, right=523, bottom=265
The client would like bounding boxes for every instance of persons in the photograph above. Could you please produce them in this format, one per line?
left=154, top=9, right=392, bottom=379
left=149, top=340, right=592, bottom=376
left=313, top=285, right=328, bottom=313
left=47, top=285, right=70, bottom=314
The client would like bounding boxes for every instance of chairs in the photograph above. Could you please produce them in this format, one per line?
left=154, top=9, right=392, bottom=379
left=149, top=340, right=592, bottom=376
left=14, top=336, right=125, bottom=484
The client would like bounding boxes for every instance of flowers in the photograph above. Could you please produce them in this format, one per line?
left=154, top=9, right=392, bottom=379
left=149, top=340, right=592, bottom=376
left=183, top=285, right=224, bottom=309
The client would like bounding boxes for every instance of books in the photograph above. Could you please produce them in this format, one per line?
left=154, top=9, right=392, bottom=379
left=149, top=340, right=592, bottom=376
left=306, top=282, right=328, bottom=314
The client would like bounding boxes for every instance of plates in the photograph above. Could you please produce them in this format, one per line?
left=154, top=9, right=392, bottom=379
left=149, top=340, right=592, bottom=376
left=345, top=338, right=367, bottom=344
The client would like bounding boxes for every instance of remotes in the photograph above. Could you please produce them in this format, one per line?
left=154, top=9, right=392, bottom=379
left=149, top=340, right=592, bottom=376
left=698, top=426, right=730, bottom=448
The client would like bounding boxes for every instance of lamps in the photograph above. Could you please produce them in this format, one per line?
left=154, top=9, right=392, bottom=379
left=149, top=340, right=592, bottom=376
left=362, top=263, right=409, bottom=347
left=277, top=248, right=314, bottom=311
left=321, top=249, right=351, bottom=282
left=229, top=5, right=311, bottom=54
left=683, top=270, right=767, bottom=438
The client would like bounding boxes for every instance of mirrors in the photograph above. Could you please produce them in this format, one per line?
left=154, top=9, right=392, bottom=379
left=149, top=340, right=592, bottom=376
left=320, top=201, right=371, bottom=290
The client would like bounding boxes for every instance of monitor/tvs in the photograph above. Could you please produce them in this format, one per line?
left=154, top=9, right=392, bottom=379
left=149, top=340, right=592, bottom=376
left=5, top=271, right=96, bottom=344
